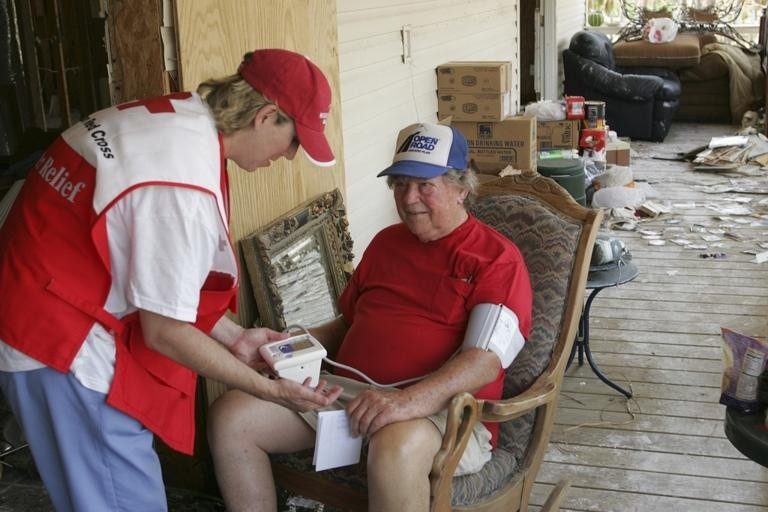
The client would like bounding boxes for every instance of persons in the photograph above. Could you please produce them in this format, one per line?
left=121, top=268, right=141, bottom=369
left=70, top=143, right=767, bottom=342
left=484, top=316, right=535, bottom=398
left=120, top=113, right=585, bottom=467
left=207, top=121, right=533, bottom=512
left=0, top=48, right=343, bottom=512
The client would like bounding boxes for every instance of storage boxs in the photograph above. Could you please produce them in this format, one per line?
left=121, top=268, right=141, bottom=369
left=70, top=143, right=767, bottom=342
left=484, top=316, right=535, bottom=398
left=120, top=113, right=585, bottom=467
left=607, top=140, right=631, bottom=166
left=434, top=60, right=581, bottom=180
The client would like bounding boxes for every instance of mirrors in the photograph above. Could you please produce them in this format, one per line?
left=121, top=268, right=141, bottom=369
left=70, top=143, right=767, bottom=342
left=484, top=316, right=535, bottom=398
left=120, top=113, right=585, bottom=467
left=236, top=186, right=357, bottom=334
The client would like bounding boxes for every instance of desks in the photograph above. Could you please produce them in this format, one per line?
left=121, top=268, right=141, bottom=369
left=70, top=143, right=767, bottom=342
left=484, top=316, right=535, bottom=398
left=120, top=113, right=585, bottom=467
left=563, top=263, right=640, bottom=398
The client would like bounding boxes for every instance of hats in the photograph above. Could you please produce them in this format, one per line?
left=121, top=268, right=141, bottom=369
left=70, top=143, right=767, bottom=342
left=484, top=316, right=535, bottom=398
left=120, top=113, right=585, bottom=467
left=376, top=122, right=468, bottom=179
left=237, top=48, right=336, bottom=167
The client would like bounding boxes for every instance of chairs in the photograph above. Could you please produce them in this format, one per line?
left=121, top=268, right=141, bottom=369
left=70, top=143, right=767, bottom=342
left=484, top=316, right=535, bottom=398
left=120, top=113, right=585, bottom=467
left=563, top=27, right=683, bottom=143
left=272, top=170, right=606, bottom=510
